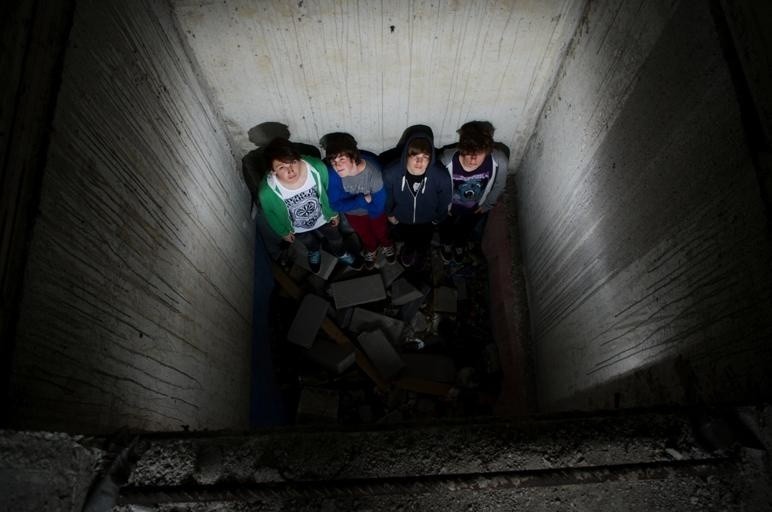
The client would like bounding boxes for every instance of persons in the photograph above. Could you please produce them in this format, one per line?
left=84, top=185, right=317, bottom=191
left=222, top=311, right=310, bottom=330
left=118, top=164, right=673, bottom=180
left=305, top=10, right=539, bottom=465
left=440, top=121, right=510, bottom=265
left=327, top=140, right=396, bottom=271
left=257, top=138, right=364, bottom=275
left=382, top=132, right=454, bottom=267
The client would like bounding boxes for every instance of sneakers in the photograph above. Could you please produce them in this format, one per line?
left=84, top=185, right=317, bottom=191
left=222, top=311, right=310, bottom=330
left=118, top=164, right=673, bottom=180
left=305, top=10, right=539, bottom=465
left=401, top=247, right=414, bottom=267
left=308, top=249, right=320, bottom=273
left=364, top=250, right=377, bottom=269
left=453, top=246, right=464, bottom=263
left=339, top=250, right=362, bottom=270
left=440, top=243, right=452, bottom=262
left=383, top=247, right=395, bottom=263
